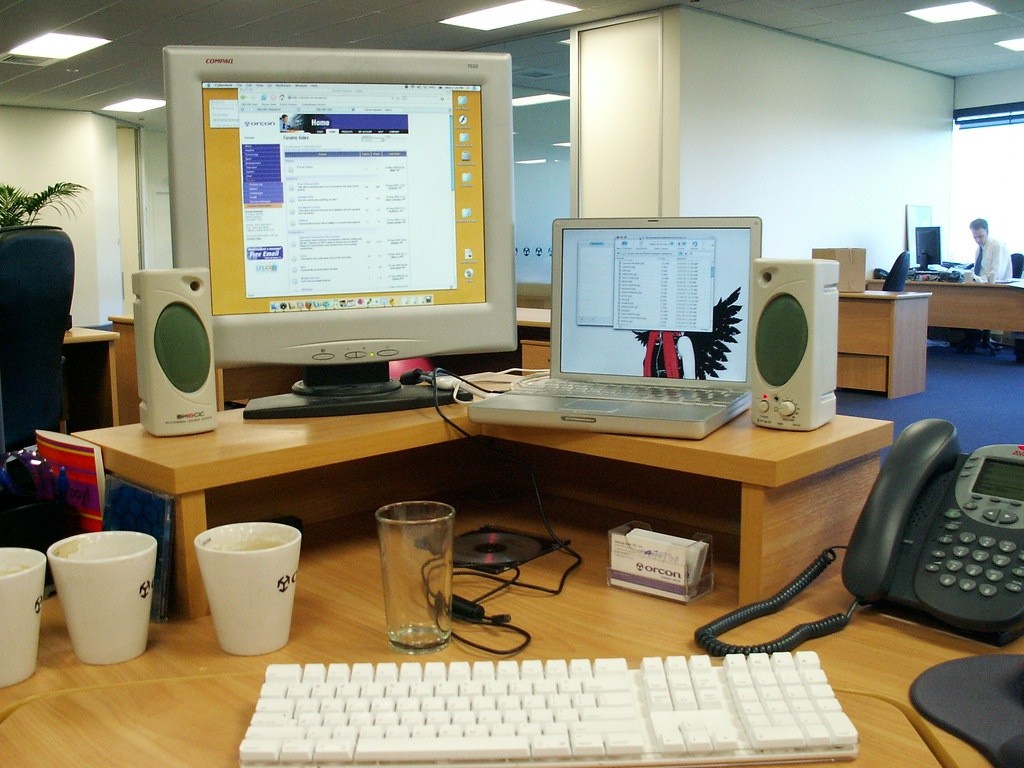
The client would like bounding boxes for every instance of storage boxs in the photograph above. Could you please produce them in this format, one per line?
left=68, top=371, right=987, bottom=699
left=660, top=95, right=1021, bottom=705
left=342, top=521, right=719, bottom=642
left=812, top=247, right=867, bottom=293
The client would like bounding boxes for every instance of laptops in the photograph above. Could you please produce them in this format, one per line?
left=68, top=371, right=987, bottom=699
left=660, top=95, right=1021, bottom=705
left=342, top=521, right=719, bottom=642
left=467, top=216, right=762, bottom=440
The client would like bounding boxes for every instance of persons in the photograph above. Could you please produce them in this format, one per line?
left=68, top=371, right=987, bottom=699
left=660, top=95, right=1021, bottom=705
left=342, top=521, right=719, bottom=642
left=949, top=219, right=1013, bottom=354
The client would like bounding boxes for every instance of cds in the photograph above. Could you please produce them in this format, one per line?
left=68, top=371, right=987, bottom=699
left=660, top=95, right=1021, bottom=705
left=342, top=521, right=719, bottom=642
left=450, top=532, right=544, bottom=563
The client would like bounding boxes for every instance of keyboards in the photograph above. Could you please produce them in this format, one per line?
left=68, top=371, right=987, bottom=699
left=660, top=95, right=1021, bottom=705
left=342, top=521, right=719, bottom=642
left=240, top=650, right=859, bottom=768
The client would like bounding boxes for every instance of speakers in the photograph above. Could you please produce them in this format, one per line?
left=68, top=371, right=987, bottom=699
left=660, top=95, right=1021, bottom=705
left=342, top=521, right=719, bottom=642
left=131, top=267, right=217, bottom=437
left=749, top=258, right=840, bottom=431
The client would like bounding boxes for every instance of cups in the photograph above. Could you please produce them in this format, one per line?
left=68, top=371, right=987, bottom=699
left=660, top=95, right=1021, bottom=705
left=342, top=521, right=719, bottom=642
left=192, top=522, right=302, bottom=654
left=377, top=500, right=456, bottom=652
left=0, top=545, right=46, bottom=689
left=46, top=531, right=157, bottom=667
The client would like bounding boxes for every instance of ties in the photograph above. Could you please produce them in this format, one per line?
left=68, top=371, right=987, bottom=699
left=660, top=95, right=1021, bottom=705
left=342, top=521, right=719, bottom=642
left=974, top=246, right=983, bottom=276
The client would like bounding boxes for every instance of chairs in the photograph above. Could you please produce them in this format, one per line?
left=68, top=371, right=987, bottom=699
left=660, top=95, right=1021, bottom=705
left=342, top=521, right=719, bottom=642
left=882, top=251, right=912, bottom=291
left=976, top=253, right=1024, bottom=357
left=1, top=226, right=74, bottom=454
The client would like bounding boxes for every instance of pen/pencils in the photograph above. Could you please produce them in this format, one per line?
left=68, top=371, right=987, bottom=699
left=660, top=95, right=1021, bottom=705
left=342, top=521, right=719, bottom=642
left=57, top=467, right=67, bottom=496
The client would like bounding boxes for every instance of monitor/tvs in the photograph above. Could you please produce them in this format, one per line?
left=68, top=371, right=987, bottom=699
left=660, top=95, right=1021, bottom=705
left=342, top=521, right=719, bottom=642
left=163, top=44, right=518, bottom=419
left=909, top=227, right=942, bottom=271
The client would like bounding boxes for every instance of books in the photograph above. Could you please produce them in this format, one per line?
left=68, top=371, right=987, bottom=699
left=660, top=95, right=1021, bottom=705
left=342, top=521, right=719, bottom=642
left=103, top=473, right=174, bottom=623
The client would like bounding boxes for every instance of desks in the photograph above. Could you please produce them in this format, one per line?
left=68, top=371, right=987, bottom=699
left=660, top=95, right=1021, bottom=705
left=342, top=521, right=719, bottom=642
left=1, top=307, right=1024, bottom=768
left=865, top=278, right=1024, bottom=332
left=836, top=289, right=934, bottom=399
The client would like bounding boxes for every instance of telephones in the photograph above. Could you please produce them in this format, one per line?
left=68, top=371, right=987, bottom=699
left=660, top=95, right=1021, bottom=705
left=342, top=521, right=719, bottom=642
left=841, top=417, right=1024, bottom=646
